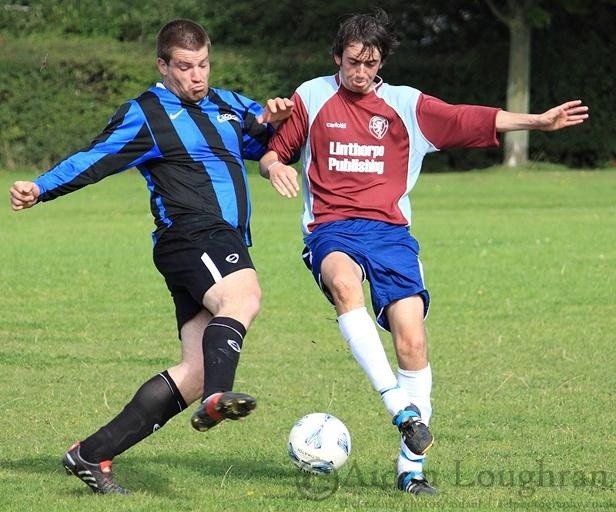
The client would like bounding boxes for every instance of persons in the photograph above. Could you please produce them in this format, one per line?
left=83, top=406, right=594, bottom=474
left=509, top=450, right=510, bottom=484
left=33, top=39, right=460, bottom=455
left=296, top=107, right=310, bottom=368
left=255, top=6, right=591, bottom=497
left=8, top=17, right=297, bottom=500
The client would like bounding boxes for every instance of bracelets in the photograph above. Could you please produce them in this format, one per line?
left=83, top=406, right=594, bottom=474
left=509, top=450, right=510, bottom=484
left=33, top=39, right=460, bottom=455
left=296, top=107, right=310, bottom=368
left=266, top=160, right=281, bottom=171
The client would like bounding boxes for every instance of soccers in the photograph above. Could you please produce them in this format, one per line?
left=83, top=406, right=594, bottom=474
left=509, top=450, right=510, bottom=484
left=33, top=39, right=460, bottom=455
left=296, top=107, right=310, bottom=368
left=286, top=413, right=351, bottom=475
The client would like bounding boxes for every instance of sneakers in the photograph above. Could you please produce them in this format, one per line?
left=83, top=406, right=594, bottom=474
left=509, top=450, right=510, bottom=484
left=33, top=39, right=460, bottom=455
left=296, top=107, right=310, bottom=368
left=395, top=470, right=440, bottom=497
left=61, top=440, right=131, bottom=495
left=391, top=401, right=434, bottom=457
left=191, top=391, right=257, bottom=432
